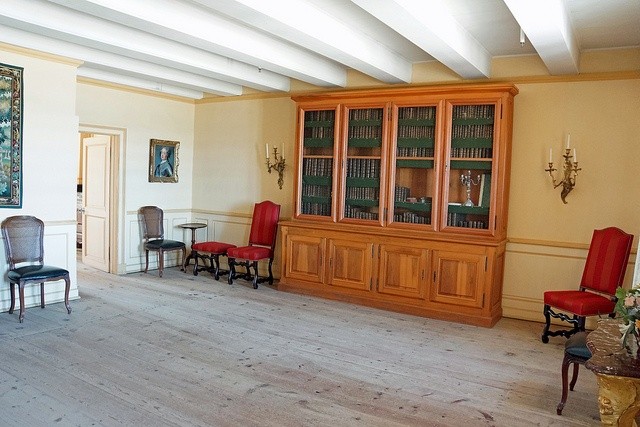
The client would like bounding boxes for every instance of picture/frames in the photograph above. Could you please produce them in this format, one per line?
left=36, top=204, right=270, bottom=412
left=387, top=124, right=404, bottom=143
left=0, top=62, right=23, bottom=208
left=148, top=138, right=181, bottom=184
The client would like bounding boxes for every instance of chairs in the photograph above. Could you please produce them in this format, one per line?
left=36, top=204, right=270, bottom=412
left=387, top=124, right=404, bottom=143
left=2, top=215, right=72, bottom=323
left=226, top=200, right=281, bottom=289
left=542, top=225, right=634, bottom=338
left=138, top=206, right=186, bottom=279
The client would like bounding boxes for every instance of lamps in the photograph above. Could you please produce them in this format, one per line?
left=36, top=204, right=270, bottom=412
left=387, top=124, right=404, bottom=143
left=265, top=148, right=286, bottom=190
left=459, top=170, right=480, bottom=207
left=545, top=148, right=581, bottom=204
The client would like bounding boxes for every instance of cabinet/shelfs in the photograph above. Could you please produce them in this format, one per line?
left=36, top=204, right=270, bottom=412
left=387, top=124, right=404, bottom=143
left=378, top=241, right=485, bottom=308
left=291, top=82, right=517, bottom=238
left=286, top=233, right=373, bottom=292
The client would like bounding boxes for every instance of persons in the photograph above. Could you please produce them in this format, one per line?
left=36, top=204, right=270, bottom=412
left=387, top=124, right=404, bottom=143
left=154, top=147, right=173, bottom=177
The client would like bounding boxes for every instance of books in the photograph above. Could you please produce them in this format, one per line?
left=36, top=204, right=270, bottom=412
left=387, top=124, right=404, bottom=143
left=453, top=105, right=493, bottom=119
left=394, top=211, right=431, bottom=224
left=396, top=148, right=433, bottom=157
left=451, top=147, right=492, bottom=158
left=399, top=107, right=436, bottom=119
left=350, top=109, right=383, bottom=120
left=309, top=110, right=334, bottom=120
left=304, top=158, right=332, bottom=176
left=348, top=159, right=379, bottom=178
left=350, top=126, right=381, bottom=138
left=346, top=186, right=378, bottom=200
left=453, top=125, right=492, bottom=138
left=395, top=184, right=410, bottom=202
left=478, top=174, right=489, bottom=207
left=447, top=212, right=484, bottom=229
left=303, top=184, right=330, bottom=197
left=345, top=204, right=378, bottom=219
left=301, top=202, right=331, bottom=216
left=312, top=127, right=334, bottom=139
left=398, top=126, right=434, bottom=138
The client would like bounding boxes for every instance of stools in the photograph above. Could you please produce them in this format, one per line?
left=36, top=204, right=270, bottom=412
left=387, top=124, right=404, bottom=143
left=194, top=241, right=237, bottom=280
left=557, top=332, right=593, bottom=418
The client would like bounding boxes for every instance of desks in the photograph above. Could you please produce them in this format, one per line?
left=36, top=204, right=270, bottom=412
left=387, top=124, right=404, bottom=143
left=586, top=317, right=639, bottom=427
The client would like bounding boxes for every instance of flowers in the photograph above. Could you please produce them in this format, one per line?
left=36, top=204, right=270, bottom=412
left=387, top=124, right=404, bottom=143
left=614, top=285, right=640, bottom=359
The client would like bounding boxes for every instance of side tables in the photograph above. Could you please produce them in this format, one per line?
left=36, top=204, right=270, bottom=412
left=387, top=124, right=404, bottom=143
left=179, top=223, right=208, bottom=276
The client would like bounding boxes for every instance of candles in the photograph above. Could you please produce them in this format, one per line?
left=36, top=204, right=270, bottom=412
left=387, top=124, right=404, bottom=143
left=266, top=143, right=269, bottom=157
left=549, top=148, right=553, bottom=161
left=282, top=143, right=285, bottom=159
left=273, top=144, right=276, bottom=148
left=567, top=134, right=570, bottom=147
left=573, top=148, right=576, bottom=161
